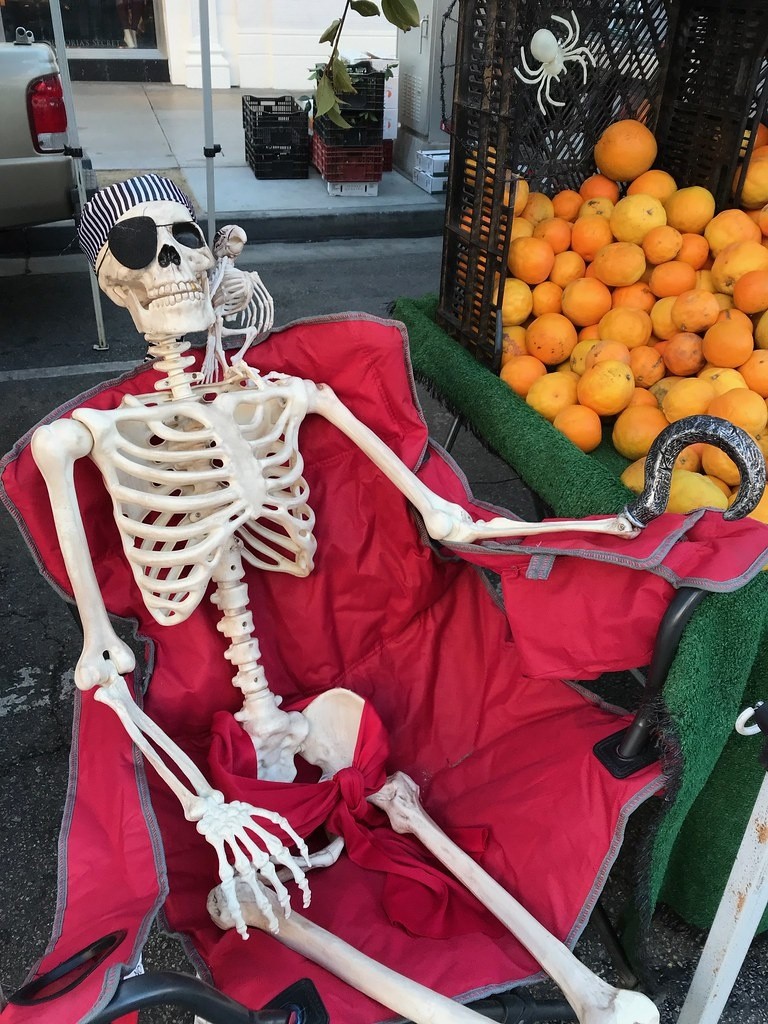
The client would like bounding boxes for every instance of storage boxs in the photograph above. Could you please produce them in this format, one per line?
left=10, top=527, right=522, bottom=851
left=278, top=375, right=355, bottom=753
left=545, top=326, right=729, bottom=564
left=435, top=0, right=768, bottom=378
left=413, top=166, right=448, bottom=195
left=416, top=149, right=449, bottom=177
left=241, top=59, right=387, bottom=197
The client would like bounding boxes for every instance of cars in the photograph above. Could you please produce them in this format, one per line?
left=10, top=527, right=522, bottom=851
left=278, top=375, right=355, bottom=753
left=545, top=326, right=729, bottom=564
left=0, top=27, right=97, bottom=234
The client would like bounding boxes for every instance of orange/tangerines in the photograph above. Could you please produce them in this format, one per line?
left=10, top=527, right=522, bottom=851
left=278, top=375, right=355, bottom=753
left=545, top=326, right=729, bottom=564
left=461, top=120, right=768, bottom=571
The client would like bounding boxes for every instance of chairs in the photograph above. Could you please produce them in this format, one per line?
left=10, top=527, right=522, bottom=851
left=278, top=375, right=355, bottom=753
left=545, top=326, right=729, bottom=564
left=0, top=309, right=768, bottom=1023
